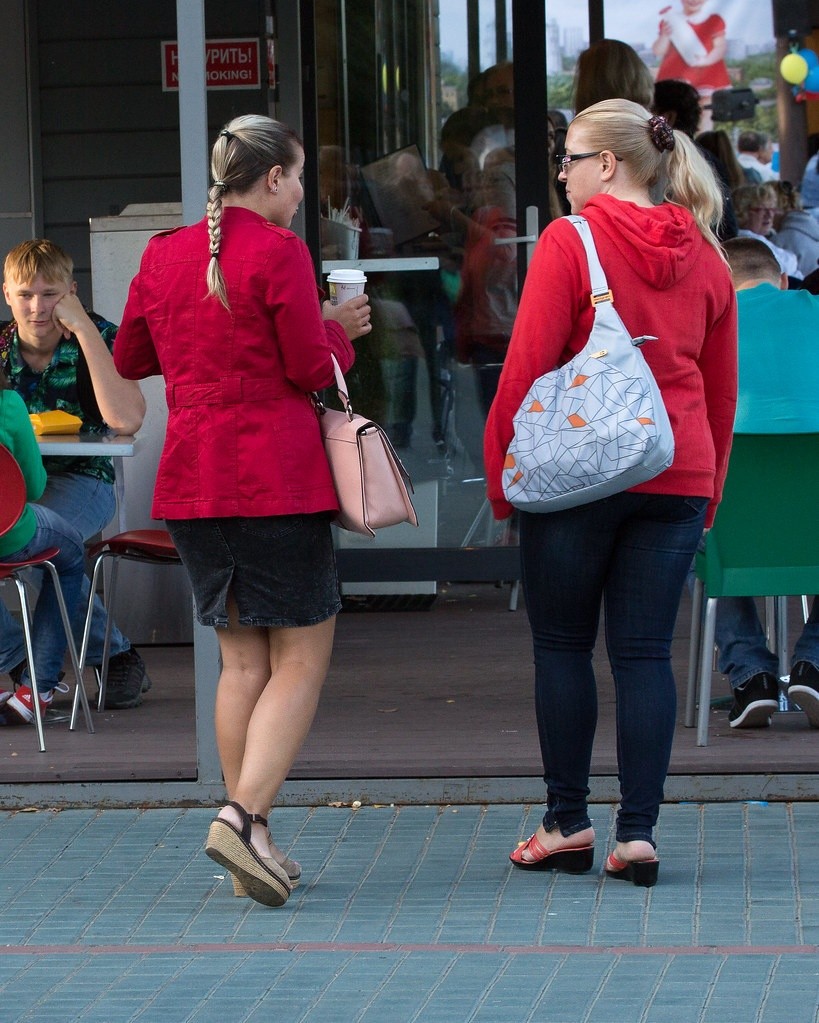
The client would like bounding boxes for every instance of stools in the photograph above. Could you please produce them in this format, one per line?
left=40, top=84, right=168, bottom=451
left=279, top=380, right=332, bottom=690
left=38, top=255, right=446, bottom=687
left=70, top=528, right=181, bottom=732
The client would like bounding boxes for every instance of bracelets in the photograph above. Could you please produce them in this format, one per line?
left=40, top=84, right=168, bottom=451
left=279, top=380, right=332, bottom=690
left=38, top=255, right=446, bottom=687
left=448, top=205, right=458, bottom=217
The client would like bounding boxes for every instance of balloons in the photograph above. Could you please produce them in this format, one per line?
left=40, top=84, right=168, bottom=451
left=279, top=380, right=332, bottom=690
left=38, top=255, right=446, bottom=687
left=780, top=48, right=819, bottom=93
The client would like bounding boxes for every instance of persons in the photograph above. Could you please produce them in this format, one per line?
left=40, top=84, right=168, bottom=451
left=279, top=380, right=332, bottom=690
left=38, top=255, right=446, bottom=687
left=484, top=64, right=568, bottom=209
left=113, top=114, right=373, bottom=908
left=738, top=129, right=781, bottom=183
left=426, top=109, right=562, bottom=423
left=0, top=345, right=84, bottom=724
left=651, top=0, right=733, bottom=132
left=720, top=180, right=819, bottom=433
left=694, top=130, right=747, bottom=194
left=573, top=39, right=655, bottom=116
left=801, top=153, right=819, bottom=209
left=355, top=134, right=465, bottom=454
left=652, top=79, right=701, bottom=136
left=0, top=240, right=148, bottom=708
left=483, top=95, right=738, bottom=888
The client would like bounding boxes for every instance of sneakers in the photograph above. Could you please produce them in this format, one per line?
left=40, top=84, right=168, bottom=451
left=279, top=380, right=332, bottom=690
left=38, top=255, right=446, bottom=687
left=8, top=683, right=70, bottom=724
left=95, top=645, right=151, bottom=709
left=728, top=673, right=778, bottom=729
left=788, top=661, right=819, bottom=731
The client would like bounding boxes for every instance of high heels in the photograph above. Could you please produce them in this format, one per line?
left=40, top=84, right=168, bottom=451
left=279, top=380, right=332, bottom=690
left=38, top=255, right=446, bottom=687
left=230, top=828, right=299, bottom=897
left=205, top=801, right=290, bottom=906
left=509, top=834, right=594, bottom=875
left=606, top=850, right=660, bottom=887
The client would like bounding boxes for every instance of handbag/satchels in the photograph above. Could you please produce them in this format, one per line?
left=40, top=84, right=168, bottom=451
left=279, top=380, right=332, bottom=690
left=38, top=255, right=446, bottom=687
left=506, top=216, right=675, bottom=513
left=312, top=352, right=419, bottom=537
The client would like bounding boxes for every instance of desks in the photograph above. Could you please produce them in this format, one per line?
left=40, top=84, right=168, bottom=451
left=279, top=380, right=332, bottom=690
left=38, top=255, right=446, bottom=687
left=35, top=431, right=138, bottom=458
left=321, top=253, right=439, bottom=275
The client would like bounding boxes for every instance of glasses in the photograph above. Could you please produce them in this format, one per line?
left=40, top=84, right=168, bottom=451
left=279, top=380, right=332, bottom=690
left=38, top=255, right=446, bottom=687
left=555, top=151, right=624, bottom=171
left=751, top=207, right=779, bottom=216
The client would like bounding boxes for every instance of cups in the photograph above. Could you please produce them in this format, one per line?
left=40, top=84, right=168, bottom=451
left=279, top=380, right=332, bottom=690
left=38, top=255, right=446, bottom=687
left=325, top=269, right=368, bottom=306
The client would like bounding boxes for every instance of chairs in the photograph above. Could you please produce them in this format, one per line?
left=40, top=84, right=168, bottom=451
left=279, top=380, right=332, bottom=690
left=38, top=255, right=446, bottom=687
left=682, top=424, right=819, bottom=747
left=0, top=443, right=94, bottom=751
